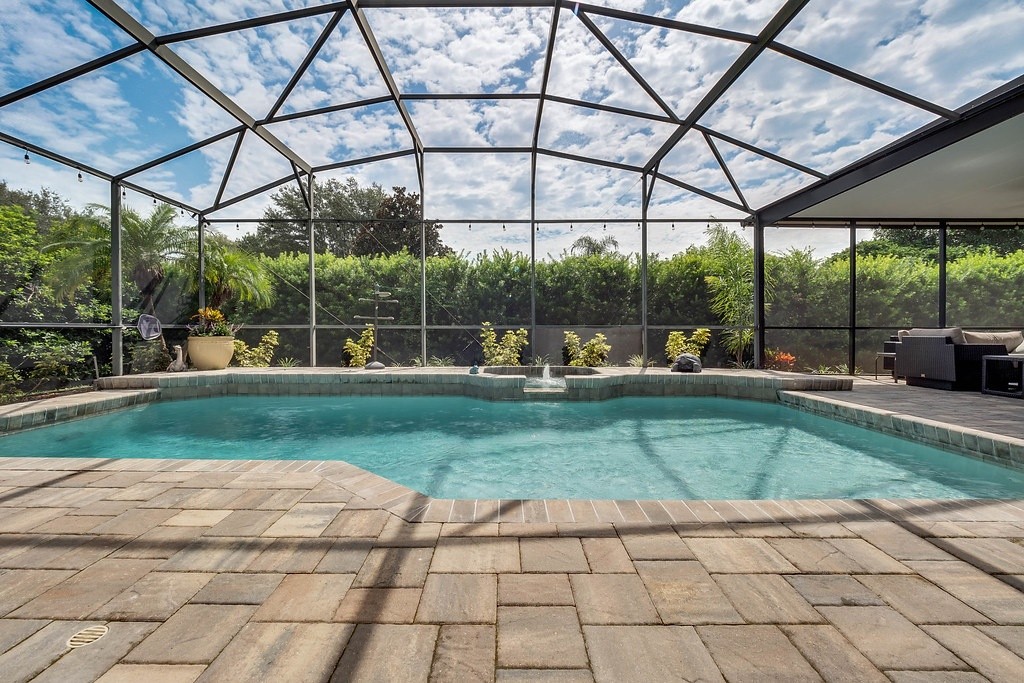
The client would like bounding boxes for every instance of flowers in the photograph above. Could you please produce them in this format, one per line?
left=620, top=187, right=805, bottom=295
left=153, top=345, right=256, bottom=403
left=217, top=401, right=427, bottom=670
left=185, top=305, right=244, bottom=336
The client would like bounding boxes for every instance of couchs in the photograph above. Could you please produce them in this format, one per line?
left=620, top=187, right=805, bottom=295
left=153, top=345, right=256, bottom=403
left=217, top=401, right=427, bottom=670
left=884, top=329, right=1024, bottom=391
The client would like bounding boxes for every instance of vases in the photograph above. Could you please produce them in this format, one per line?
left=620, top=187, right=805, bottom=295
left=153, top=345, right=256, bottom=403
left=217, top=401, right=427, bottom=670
left=187, top=336, right=234, bottom=371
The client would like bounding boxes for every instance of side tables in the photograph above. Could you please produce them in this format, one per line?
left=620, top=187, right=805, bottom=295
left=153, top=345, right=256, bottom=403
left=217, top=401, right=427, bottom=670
left=875, top=352, right=898, bottom=384
left=981, top=353, right=1024, bottom=399
